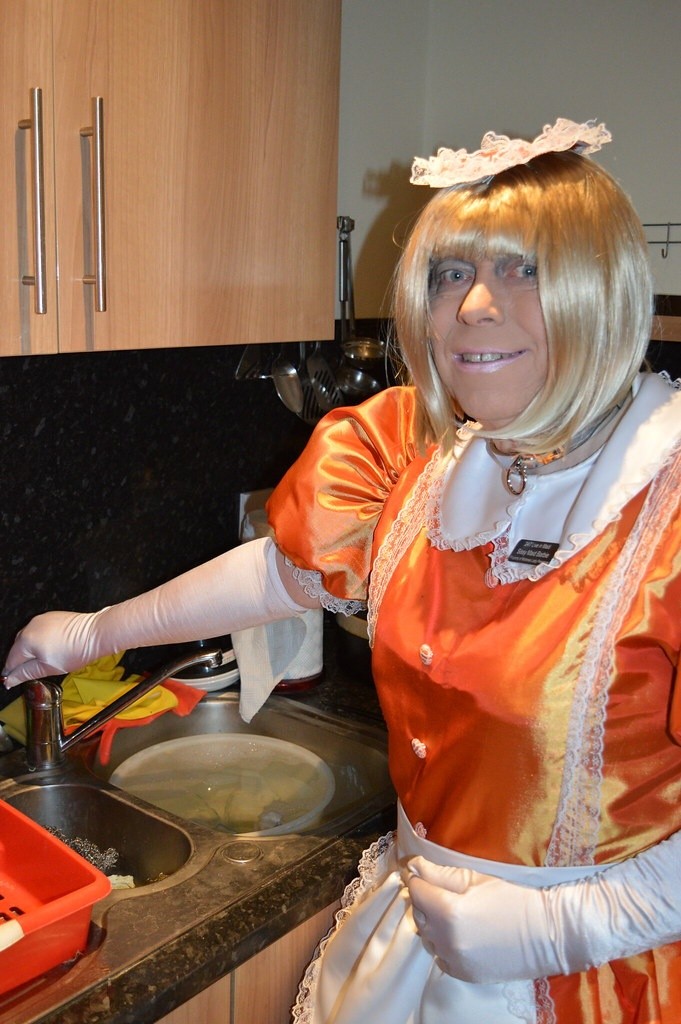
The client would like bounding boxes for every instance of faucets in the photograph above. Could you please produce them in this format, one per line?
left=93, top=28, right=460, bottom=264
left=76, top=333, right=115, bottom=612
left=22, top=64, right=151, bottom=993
left=23, top=650, right=226, bottom=779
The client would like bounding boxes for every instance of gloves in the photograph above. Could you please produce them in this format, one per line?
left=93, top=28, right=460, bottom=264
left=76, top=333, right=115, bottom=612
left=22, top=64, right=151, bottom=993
left=405, top=827, right=680, bottom=985
left=1, top=535, right=311, bottom=690
left=0, top=649, right=208, bottom=766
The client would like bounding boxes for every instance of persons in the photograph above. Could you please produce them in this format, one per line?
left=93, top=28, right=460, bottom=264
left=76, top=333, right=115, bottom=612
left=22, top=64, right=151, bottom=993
left=2, top=146, right=679, bottom=1023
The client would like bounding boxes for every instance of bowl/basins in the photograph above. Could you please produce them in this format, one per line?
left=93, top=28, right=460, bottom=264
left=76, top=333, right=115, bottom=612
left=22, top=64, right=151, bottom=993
left=108, top=734, right=335, bottom=837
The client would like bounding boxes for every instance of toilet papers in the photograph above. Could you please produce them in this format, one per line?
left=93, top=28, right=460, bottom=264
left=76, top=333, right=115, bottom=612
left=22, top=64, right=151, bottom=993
left=236, top=494, right=325, bottom=725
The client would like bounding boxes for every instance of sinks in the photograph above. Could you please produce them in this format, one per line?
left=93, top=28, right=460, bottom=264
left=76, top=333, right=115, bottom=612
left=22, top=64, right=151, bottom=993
left=80, top=686, right=391, bottom=846
left=1, top=768, right=218, bottom=1024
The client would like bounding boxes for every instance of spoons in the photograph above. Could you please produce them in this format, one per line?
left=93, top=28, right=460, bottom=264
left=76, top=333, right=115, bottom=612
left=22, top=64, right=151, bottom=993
left=271, top=345, right=305, bottom=414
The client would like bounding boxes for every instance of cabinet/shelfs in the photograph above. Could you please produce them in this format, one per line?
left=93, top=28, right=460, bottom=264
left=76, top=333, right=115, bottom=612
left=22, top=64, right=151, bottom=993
left=0, top=0, right=342, bottom=358
